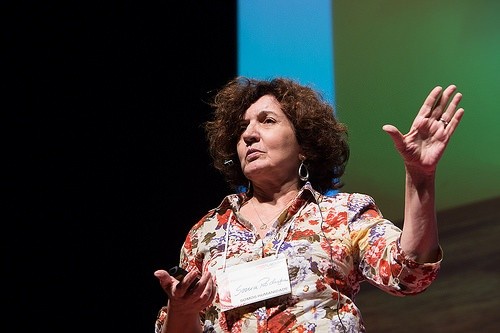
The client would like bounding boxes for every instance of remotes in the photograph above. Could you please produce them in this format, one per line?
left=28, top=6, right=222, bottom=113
left=169, top=266, right=200, bottom=290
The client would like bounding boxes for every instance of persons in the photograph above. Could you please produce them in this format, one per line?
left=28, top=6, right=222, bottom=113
left=154, top=77, right=465, bottom=333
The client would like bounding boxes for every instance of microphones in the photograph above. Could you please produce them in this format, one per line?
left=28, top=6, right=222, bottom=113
left=223, top=159, right=234, bottom=168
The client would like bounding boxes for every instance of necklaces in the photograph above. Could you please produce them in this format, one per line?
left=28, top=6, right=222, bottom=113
left=250, top=199, right=292, bottom=229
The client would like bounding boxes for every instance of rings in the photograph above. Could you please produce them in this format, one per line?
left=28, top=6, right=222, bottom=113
left=439, top=118, right=449, bottom=126
